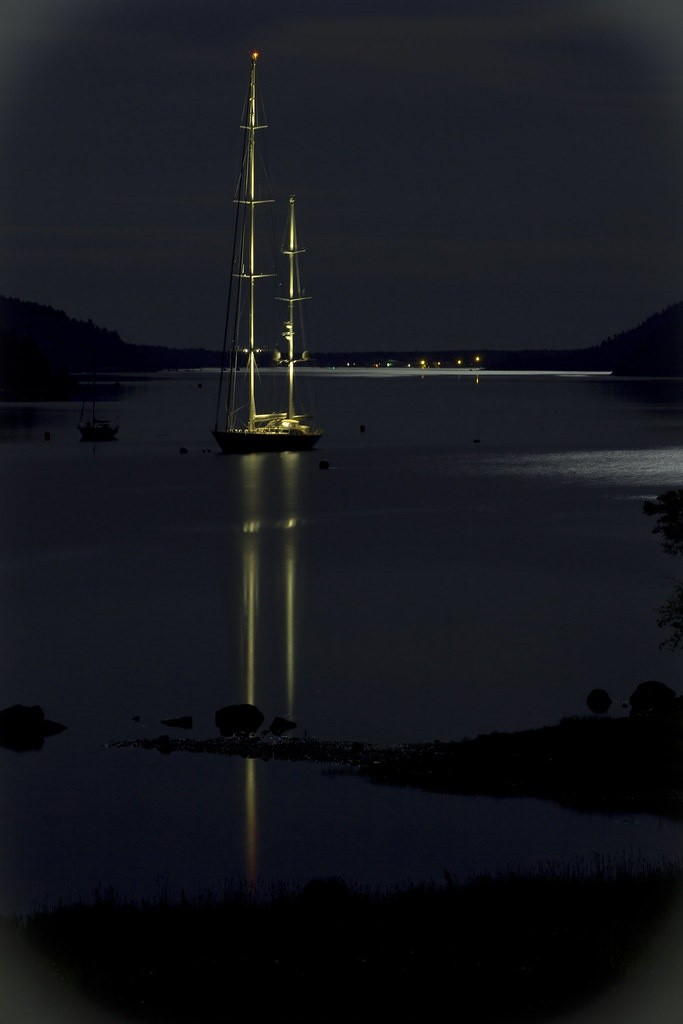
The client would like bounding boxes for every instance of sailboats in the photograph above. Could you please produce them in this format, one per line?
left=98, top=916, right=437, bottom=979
left=76, top=347, right=120, bottom=442
left=210, top=50, right=324, bottom=453
